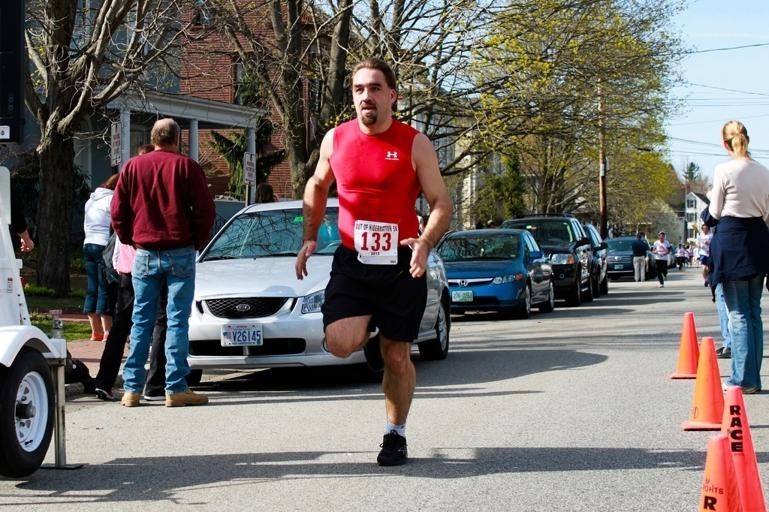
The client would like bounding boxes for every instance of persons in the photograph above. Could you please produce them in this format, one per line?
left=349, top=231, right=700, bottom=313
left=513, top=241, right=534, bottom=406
left=632, top=222, right=713, bottom=288
left=82, top=118, right=216, bottom=406
left=702, top=121, right=768, bottom=395
left=294, top=60, right=453, bottom=467
left=0, top=167, right=36, bottom=325
left=703, top=281, right=731, bottom=359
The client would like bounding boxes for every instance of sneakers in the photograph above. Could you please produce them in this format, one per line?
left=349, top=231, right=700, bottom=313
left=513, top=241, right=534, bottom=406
left=91, top=332, right=109, bottom=341
left=716, top=347, right=732, bottom=358
left=722, top=382, right=762, bottom=394
left=165, top=390, right=210, bottom=408
left=94, top=387, right=119, bottom=402
left=122, top=391, right=140, bottom=407
left=144, top=386, right=165, bottom=400
left=377, top=429, right=409, bottom=466
left=363, top=332, right=384, bottom=371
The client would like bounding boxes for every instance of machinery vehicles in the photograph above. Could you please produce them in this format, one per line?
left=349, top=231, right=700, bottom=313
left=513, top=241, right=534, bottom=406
left=1, top=161, right=83, bottom=486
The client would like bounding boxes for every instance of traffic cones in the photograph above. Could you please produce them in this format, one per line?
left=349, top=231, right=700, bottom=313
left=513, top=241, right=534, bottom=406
left=668, top=310, right=703, bottom=380
left=720, top=382, right=769, bottom=512
left=695, top=432, right=743, bottom=512
left=679, top=335, right=726, bottom=433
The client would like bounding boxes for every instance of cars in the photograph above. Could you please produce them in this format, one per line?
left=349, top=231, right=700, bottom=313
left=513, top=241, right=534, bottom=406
left=600, top=235, right=678, bottom=281
left=189, top=194, right=461, bottom=383
left=432, top=222, right=560, bottom=318
left=584, top=221, right=612, bottom=298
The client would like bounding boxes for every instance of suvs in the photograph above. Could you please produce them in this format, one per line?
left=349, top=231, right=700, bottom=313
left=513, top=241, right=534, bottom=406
left=490, top=208, right=598, bottom=306
